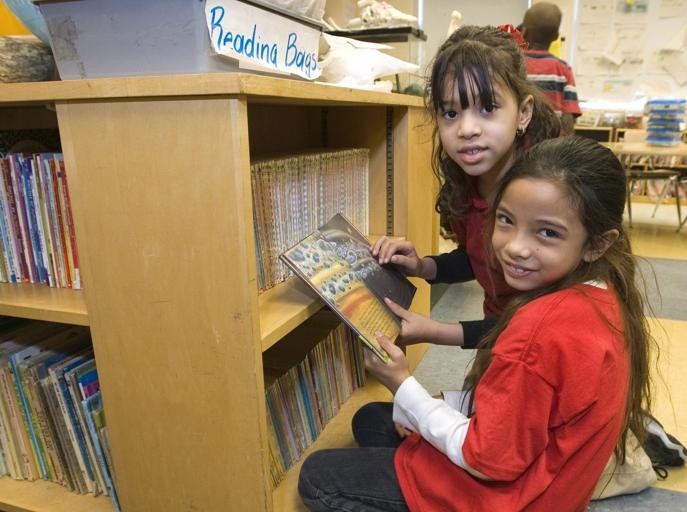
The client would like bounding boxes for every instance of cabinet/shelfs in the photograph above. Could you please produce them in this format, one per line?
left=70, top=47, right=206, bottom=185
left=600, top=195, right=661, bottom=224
left=1, top=72, right=437, bottom=512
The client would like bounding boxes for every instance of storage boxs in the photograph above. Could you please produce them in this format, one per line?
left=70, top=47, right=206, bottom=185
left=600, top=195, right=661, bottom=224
left=32, top=0, right=324, bottom=82
left=643, top=100, right=687, bottom=148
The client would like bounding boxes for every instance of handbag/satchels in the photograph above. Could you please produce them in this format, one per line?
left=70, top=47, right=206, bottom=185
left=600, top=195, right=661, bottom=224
left=591, top=411, right=668, bottom=500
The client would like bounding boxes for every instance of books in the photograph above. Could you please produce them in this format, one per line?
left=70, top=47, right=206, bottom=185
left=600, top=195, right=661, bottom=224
left=0, top=316, right=120, bottom=511
left=263, top=306, right=371, bottom=488
left=252, top=145, right=370, bottom=294
left=278, top=212, right=418, bottom=362
left=0, top=151, right=82, bottom=290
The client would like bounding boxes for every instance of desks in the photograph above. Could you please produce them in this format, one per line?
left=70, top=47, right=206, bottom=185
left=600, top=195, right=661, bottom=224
left=575, top=127, right=687, bottom=232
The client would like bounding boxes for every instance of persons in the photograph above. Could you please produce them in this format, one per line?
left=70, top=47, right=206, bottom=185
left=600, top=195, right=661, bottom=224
left=506, top=3, right=583, bottom=127
left=297, top=132, right=655, bottom=511
left=368, top=25, right=686, bottom=502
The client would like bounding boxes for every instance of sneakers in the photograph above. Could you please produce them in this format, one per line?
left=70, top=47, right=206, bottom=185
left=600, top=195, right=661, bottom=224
left=630, top=411, right=687, bottom=467
